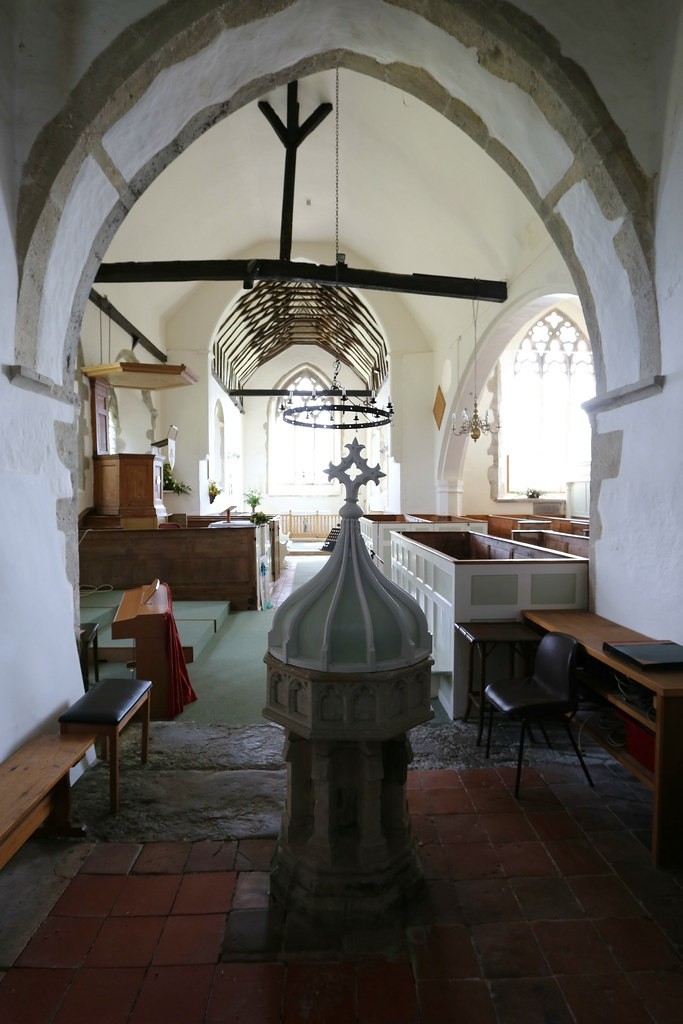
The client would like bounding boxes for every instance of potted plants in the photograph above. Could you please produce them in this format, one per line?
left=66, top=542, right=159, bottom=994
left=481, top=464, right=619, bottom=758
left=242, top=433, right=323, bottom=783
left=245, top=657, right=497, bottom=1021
left=242, top=489, right=263, bottom=513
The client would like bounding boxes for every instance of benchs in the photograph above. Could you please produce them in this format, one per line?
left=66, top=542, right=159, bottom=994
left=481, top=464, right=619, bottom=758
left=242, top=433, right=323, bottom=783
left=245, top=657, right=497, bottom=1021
left=463, top=512, right=590, bottom=557
left=0, top=733, right=100, bottom=874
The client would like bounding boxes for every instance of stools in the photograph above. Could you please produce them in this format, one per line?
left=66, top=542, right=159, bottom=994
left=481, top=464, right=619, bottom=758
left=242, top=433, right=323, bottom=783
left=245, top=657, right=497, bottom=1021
left=80, top=622, right=100, bottom=692
left=57, top=677, right=155, bottom=818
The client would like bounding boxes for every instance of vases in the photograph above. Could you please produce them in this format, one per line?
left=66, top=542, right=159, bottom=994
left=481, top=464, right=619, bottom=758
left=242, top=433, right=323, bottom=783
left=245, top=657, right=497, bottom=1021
left=528, top=493, right=540, bottom=498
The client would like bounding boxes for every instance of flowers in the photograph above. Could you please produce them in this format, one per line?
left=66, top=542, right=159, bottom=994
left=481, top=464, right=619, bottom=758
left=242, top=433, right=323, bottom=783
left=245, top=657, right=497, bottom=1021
left=208, top=479, right=222, bottom=497
left=249, top=511, right=273, bottom=533
left=164, top=463, right=192, bottom=496
left=516, top=487, right=548, bottom=498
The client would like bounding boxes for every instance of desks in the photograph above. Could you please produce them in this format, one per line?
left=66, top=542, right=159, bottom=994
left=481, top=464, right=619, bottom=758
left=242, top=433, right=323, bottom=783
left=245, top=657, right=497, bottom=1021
left=521, top=608, right=683, bottom=872
left=453, top=621, right=544, bottom=747
left=207, top=520, right=257, bottom=528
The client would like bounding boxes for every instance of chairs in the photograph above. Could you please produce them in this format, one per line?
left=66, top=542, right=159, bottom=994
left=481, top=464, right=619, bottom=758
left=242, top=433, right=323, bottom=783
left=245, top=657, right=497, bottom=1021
left=484, top=631, right=596, bottom=800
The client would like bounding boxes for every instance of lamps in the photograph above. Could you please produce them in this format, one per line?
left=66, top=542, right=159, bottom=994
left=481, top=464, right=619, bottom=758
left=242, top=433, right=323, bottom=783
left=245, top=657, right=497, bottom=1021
left=451, top=299, right=502, bottom=443
left=277, top=65, right=396, bottom=430
